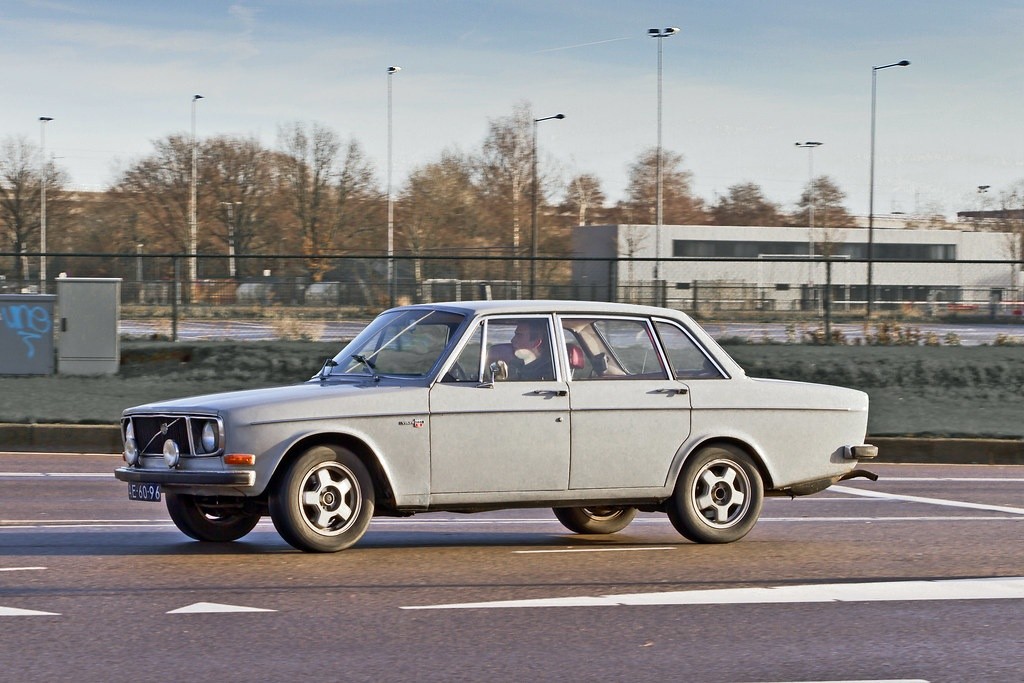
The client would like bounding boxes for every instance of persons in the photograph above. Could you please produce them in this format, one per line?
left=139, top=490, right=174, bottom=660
left=510, top=321, right=554, bottom=380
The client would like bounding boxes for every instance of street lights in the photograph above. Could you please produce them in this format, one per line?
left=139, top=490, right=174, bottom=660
left=190, top=94, right=204, bottom=303
left=385, top=66, right=400, bottom=285
left=867, top=60, right=910, bottom=321
left=648, top=27, right=680, bottom=281
left=529, top=114, right=566, bottom=296
left=795, top=140, right=823, bottom=310
left=38, top=117, right=54, bottom=292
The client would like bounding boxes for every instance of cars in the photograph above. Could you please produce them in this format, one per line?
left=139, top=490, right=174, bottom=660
left=113, top=299, right=878, bottom=553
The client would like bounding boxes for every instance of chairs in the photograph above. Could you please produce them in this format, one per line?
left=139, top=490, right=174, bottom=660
left=566, top=343, right=584, bottom=377
left=488, top=343, right=514, bottom=363
